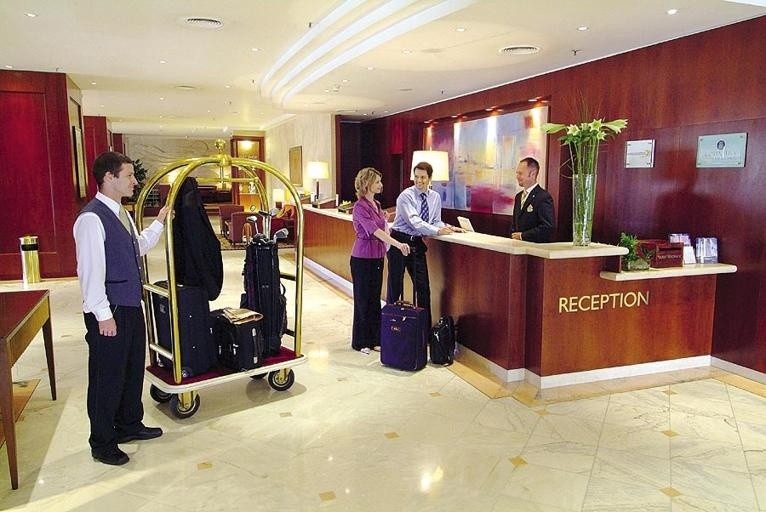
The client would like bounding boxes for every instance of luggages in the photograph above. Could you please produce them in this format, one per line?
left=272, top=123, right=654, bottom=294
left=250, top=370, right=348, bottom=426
left=380, top=246, right=429, bottom=371
left=154, top=282, right=216, bottom=378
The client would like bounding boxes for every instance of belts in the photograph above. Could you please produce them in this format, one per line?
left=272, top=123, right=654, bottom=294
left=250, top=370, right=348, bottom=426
left=393, top=231, right=422, bottom=242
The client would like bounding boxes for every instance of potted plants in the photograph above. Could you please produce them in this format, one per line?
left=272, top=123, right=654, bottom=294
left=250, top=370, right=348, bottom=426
left=621, top=231, right=650, bottom=272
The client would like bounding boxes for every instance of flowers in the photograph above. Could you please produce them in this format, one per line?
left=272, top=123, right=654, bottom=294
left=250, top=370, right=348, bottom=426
left=541, top=91, right=629, bottom=174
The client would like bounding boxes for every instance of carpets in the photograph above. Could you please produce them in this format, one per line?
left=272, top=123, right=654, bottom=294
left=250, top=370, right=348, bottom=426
left=216, top=232, right=297, bottom=251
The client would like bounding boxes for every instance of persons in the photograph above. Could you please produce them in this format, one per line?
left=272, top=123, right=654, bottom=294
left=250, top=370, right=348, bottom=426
left=386, top=161, right=469, bottom=350
left=510, top=158, right=555, bottom=243
left=351, top=166, right=410, bottom=353
left=73, top=151, right=176, bottom=465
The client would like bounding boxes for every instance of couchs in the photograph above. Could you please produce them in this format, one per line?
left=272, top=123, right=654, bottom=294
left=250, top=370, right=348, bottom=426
left=225, top=212, right=263, bottom=248
left=271, top=205, right=297, bottom=245
left=219, top=205, right=244, bottom=235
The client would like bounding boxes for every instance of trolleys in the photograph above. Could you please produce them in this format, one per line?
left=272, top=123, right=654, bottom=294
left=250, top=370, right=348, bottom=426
left=137, top=142, right=305, bottom=418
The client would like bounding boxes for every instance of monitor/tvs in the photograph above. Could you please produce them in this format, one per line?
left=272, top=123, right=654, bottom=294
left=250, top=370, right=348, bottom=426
left=458, top=217, right=474, bottom=232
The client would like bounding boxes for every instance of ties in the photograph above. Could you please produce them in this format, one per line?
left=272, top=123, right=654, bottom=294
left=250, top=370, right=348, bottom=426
left=521, top=191, right=528, bottom=210
left=420, top=194, right=429, bottom=224
left=120, top=205, right=131, bottom=234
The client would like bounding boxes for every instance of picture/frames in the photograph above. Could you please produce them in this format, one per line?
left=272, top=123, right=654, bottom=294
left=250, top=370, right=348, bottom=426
left=73, top=124, right=90, bottom=201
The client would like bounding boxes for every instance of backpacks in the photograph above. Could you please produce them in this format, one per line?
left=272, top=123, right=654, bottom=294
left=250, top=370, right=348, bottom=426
left=431, top=316, right=460, bottom=366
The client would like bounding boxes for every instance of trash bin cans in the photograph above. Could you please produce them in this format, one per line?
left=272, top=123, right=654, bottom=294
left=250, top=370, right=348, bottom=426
left=20, top=237, right=40, bottom=283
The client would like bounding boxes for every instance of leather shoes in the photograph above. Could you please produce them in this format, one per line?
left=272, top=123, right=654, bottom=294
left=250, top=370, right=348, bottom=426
left=361, top=347, right=369, bottom=353
left=374, top=346, right=381, bottom=351
left=92, top=444, right=129, bottom=465
left=116, top=426, right=162, bottom=444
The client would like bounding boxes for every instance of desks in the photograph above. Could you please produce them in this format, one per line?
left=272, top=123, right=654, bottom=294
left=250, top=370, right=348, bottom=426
left=0, top=291, right=61, bottom=493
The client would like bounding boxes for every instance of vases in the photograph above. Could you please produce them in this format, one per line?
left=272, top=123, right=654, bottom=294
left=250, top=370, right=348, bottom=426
left=571, top=173, right=596, bottom=247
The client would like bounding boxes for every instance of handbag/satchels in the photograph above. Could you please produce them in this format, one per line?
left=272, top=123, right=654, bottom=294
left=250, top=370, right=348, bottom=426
left=430, top=315, right=455, bottom=364
left=213, top=308, right=261, bottom=372
left=240, top=240, right=286, bottom=356
